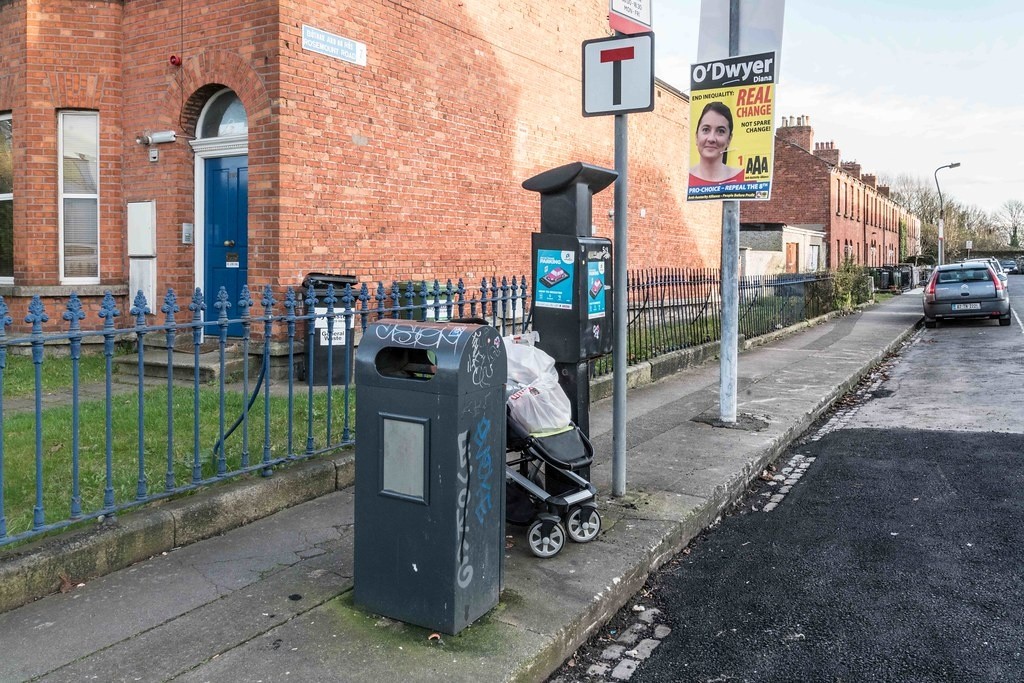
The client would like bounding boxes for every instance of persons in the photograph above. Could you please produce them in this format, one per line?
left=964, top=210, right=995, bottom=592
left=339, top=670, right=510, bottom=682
left=689, top=102, right=744, bottom=188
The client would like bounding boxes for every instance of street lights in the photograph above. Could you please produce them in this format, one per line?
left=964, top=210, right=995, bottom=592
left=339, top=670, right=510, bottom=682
left=935, top=161, right=961, bottom=266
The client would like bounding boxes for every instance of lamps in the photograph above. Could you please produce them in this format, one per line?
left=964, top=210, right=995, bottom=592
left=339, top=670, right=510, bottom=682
left=135, top=135, right=152, bottom=146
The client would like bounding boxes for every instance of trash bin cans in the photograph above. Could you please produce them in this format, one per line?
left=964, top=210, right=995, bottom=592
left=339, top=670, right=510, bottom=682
left=301, top=272, right=361, bottom=386
left=892, top=267, right=902, bottom=287
left=354, top=319, right=509, bottom=636
left=879, top=270, right=891, bottom=289
left=397, top=280, right=459, bottom=366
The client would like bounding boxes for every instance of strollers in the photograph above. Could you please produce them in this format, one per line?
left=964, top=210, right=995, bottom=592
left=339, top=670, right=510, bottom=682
left=453, top=318, right=603, bottom=558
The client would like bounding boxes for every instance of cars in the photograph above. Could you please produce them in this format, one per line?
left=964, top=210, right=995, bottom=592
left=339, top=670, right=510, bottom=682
left=999, top=259, right=1018, bottom=274
left=959, top=256, right=1008, bottom=290
left=919, top=261, right=1012, bottom=328
